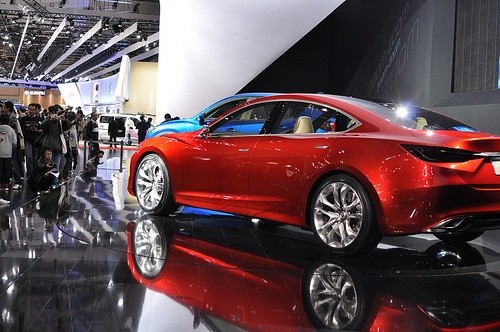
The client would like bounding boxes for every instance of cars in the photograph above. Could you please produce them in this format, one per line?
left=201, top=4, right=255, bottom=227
left=126, top=92, right=500, bottom=257
left=145, top=93, right=336, bottom=140
left=124, top=214, right=499, bottom=331
left=172, top=205, right=235, bottom=217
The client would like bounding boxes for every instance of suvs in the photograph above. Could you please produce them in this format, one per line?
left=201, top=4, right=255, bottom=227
left=96, top=112, right=154, bottom=146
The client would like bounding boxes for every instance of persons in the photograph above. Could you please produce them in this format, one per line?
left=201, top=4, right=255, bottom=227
left=135, top=115, right=152, bottom=144
left=0, top=101, right=103, bottom=205
left=0, top=176, right=127, bottom=247
left=108, top=116, right=119, bottom=148
left=165, top=114, right=180, bottom=121
left=115, top=117, right=125, bottom=137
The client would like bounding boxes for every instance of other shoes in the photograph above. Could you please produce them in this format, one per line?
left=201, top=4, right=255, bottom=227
left=5, top=187, right=9, bottom=190
left=37, top=191, right=40, bottom=195
left=0, top=198, right=10, bottom=204
left=0, top=186, right=4, bottom=190
left=12, top=184, right=23, bottom=189
left=43, top=191, right=48, bottom=193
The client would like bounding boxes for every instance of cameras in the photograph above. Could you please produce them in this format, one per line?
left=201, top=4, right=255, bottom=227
left=56, top=116, right=61, bottom=120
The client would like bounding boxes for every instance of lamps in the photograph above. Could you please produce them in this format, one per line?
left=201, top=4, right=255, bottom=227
left=0, top=0, right=147, bottom=83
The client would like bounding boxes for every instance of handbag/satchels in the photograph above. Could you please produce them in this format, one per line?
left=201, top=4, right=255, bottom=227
left=60, top=119, right=68, bottom=154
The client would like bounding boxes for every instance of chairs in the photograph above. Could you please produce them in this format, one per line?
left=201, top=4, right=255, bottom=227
left=291, top=115, right=314, bottom=133
